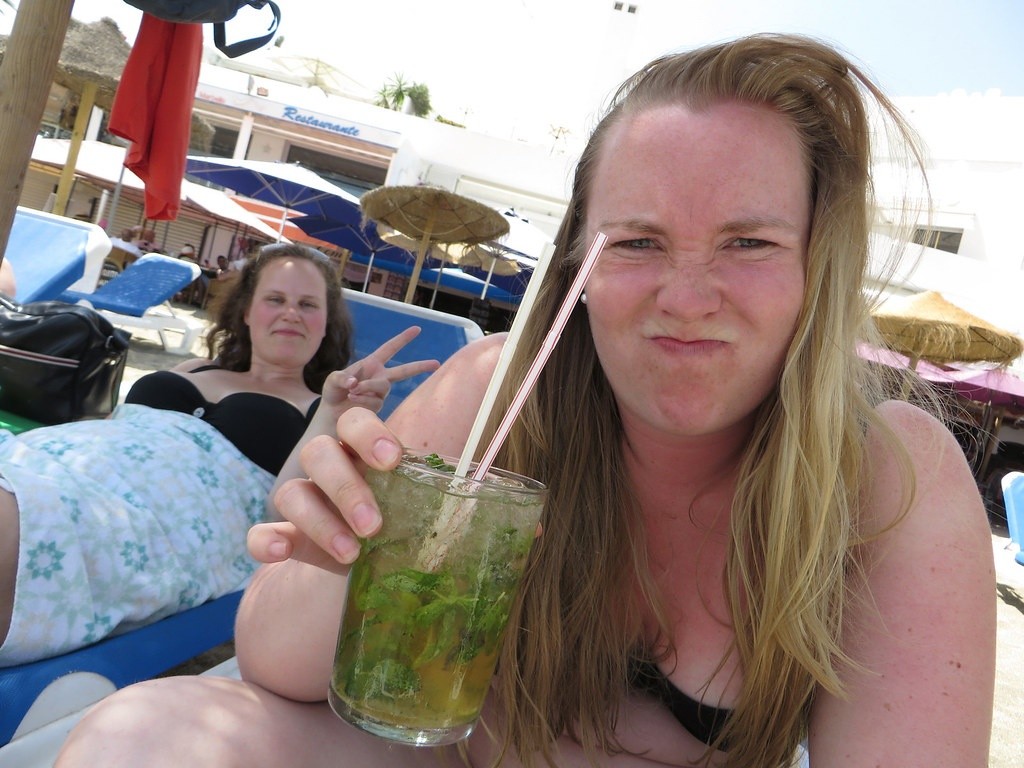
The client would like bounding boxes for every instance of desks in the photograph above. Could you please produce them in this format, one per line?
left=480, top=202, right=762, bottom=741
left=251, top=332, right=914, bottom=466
left=139, top=247, right=219, bottom=280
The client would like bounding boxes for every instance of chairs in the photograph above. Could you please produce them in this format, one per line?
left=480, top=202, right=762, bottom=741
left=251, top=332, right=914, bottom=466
left=1001, top=472, right=1024, bottom=565
left=0, top=204, right=232, bottom=353
left=0, top=285, right=485, bottom=746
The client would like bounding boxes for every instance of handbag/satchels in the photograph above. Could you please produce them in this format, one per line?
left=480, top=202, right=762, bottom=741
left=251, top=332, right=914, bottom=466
left=0, top=294, right=133, bottom=425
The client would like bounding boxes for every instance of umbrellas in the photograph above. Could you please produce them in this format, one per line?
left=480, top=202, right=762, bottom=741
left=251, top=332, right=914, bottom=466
left=184, top=134, right=361, bottom=257
left=286, top=181, right=550, bottom=326
left=859, top=290, right=1024, bottom=402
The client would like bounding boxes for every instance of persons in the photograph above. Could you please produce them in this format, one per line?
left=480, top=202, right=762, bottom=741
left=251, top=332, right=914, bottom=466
left=51, top=33, right=994, bottom=768
left=96, top=220, right=250, bottom=276
left=0, top=243, right=440, bottom=671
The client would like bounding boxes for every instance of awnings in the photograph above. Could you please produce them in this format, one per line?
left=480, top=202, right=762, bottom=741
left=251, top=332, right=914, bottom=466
left=24, top=128, right=297, bottom=245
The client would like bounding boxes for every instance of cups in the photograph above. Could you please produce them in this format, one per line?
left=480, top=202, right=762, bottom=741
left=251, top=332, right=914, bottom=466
left=324, top=452, right=548, bottom=747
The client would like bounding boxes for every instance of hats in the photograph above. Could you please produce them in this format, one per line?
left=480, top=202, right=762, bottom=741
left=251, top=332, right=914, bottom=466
left=181, top=246, right=193, bottom=254
